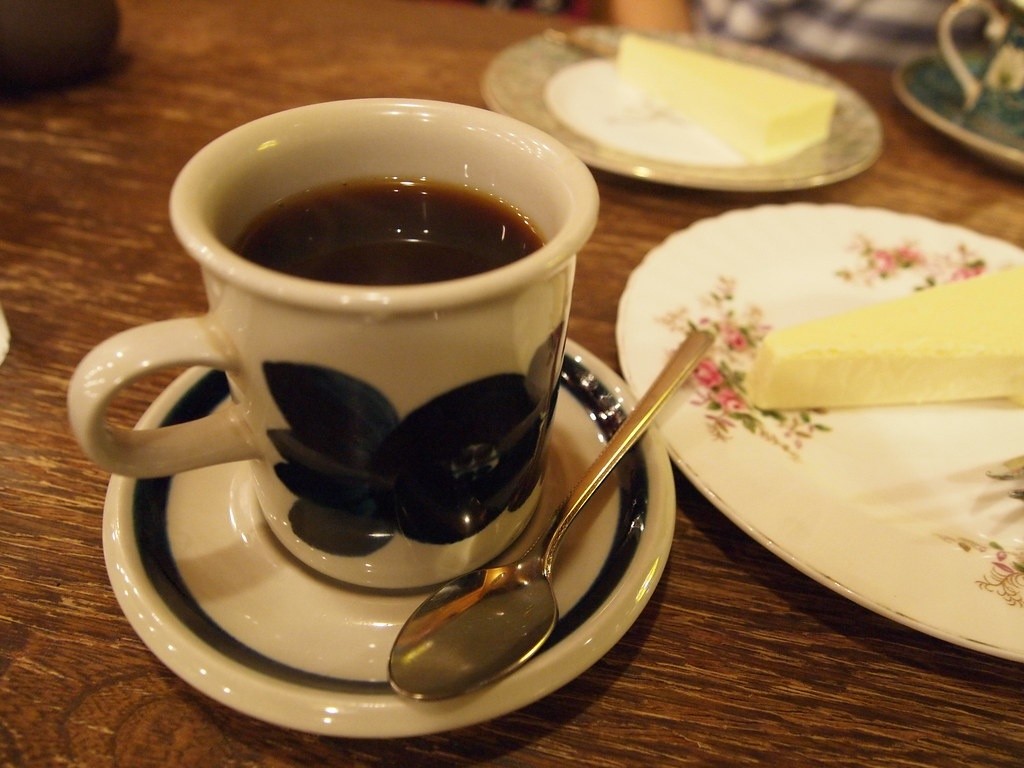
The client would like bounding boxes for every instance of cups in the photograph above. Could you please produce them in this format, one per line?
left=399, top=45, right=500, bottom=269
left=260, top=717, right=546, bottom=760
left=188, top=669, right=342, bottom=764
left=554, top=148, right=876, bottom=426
left=936, top=0, right=1024, bottom=152
left=66, top=96, right=602, bottom=598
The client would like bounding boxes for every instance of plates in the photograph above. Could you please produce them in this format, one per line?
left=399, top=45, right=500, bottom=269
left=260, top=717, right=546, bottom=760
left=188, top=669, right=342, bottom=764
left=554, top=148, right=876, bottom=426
left=101, top=336, right=677, bottom=740
left=478, top=24, right=884, bottom=192
left=611, top=200, right=1024, bottom=664
left=890, top=50, right=1024, bottom=173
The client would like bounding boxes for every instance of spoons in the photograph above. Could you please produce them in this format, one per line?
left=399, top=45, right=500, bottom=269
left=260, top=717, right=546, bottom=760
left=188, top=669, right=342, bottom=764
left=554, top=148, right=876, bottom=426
left=385, top=329, right=715, bottom=703
